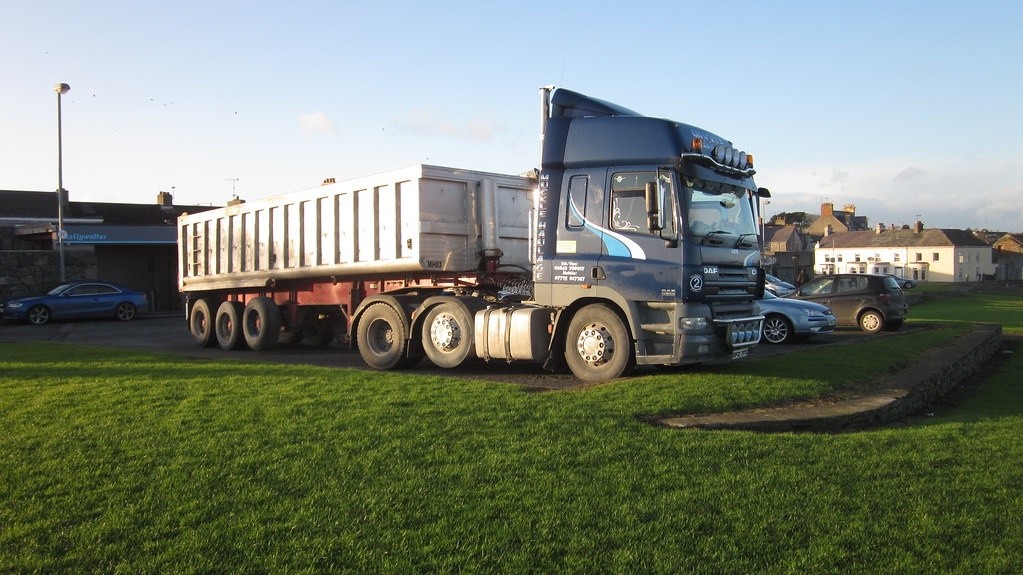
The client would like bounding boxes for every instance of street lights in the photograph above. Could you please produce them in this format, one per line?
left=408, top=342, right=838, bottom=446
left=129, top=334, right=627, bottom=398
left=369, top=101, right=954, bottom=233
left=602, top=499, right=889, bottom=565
left=761, top=200, right=771, bottom=265
left=52, top=82, right=70, bottom=284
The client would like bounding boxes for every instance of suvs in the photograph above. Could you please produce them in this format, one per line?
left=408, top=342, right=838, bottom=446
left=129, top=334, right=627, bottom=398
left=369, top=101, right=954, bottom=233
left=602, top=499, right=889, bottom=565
left=778, top=273, right=908, bottom=333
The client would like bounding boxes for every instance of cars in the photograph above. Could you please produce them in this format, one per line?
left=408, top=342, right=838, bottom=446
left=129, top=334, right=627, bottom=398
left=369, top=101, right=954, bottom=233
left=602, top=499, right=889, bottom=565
left=891, top=274, right=916, bottom=290
left=2, top=281, right=152, bottom=326
left=765, top=272, right=796, bottom=298
left=755, top=288, right=836, bottom=345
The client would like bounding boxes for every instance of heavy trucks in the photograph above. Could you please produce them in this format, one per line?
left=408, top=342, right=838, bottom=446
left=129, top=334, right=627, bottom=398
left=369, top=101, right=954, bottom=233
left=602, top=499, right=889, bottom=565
left=176, top=85, right=767, bottom=384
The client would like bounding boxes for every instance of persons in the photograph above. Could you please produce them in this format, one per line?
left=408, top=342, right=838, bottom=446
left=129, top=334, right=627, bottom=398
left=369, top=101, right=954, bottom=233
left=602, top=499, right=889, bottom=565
left=796, top=268, right=810, bottom=287
